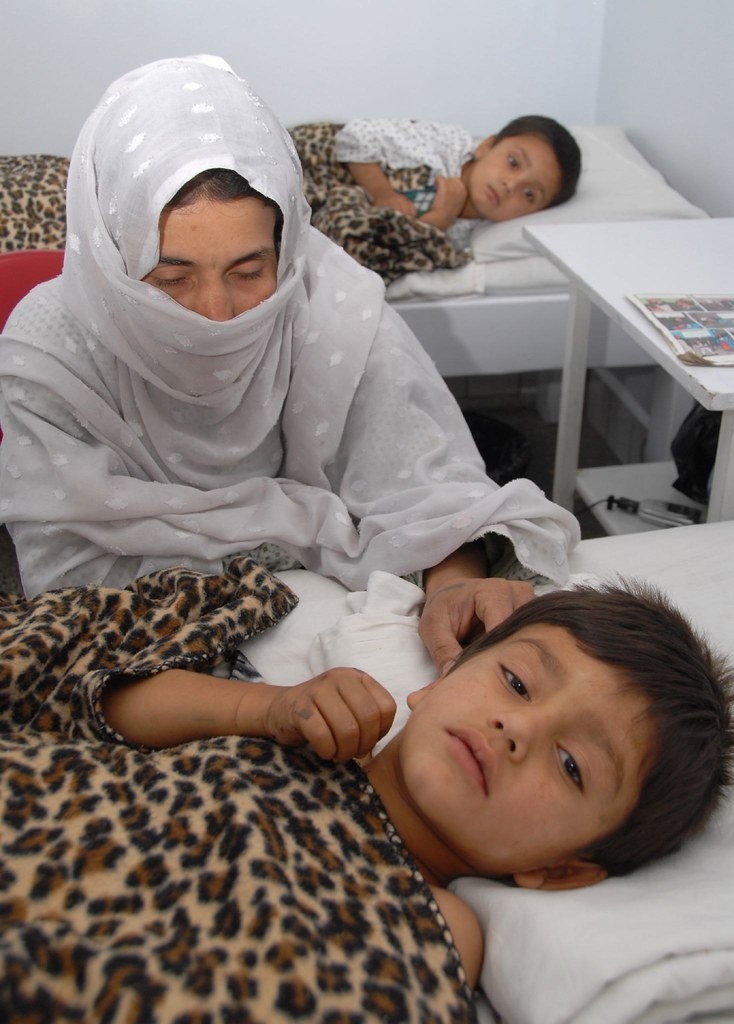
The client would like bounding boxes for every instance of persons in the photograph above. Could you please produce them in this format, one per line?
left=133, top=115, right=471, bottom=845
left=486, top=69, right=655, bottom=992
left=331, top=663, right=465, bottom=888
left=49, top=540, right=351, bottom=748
left=103, top=573, right=734, bottom=1000
left=0, top=54, right=583, bottom=676
left=333, top=115, right=580, bottom=244
left=644, top=296, right=734, bottom=358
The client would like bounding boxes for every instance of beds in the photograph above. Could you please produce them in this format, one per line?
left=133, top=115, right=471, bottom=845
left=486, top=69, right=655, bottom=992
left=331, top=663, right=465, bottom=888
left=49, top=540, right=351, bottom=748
left=386, top=121, right=693, bottom=463
left=237, top=522, right=734, bottom=1024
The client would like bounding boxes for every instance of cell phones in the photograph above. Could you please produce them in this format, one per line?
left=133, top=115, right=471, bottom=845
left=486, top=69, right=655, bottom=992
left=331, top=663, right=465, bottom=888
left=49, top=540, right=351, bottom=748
left=638, top=498, right=701, bottom=527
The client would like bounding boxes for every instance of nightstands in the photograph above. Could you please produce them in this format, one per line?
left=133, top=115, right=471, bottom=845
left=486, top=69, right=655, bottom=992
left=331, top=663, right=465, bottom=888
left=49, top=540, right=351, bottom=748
left=522, top=212, right=734, bottom=537
